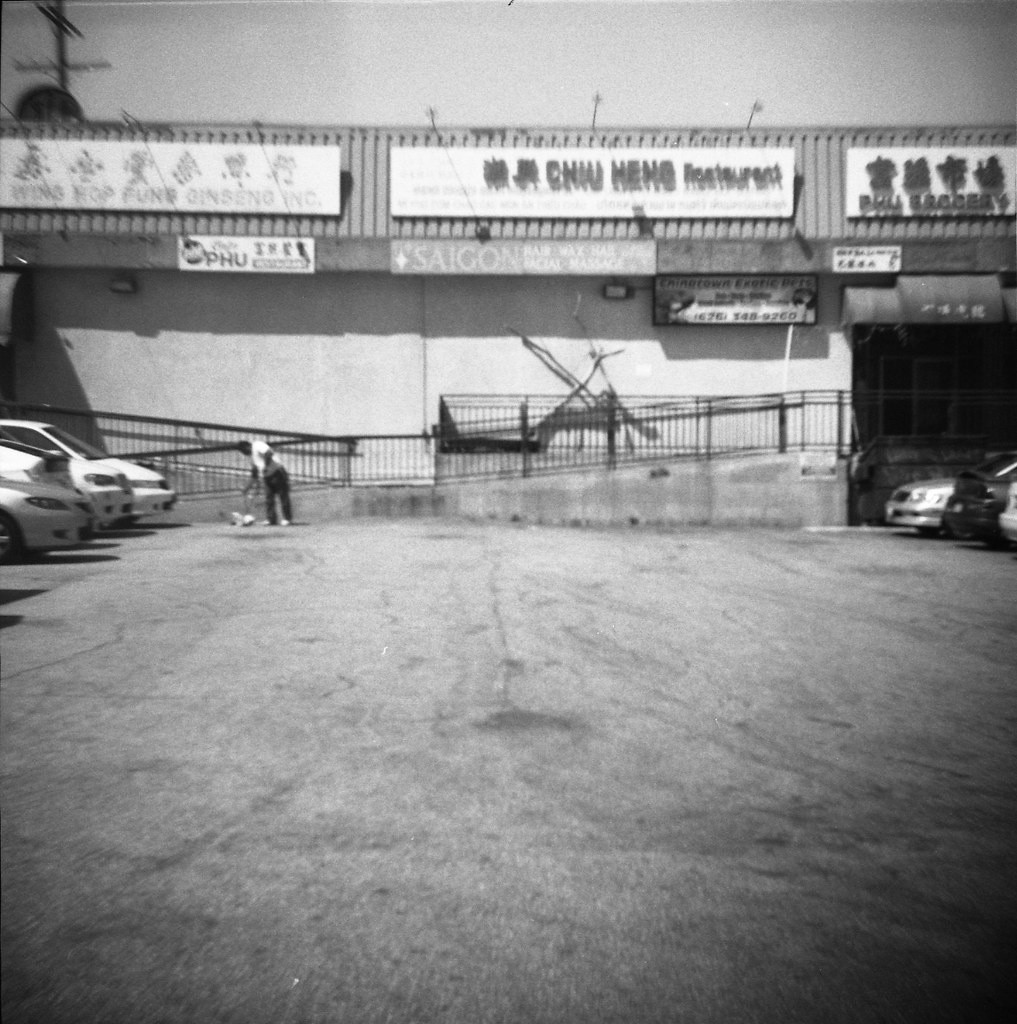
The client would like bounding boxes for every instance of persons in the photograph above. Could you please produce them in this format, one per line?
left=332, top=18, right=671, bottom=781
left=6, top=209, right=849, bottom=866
left=237, top=440, right=292, bottom=526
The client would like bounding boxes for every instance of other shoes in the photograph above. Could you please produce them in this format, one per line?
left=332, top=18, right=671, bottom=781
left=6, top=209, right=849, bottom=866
left=261, top=519, right=271, bottom=525
left=281, top=519, right=291, bottom=525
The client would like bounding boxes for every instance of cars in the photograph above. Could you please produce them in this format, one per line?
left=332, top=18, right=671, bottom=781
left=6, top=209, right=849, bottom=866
left=0, top=419, right=177, bottom=536
left=0, top=438, right=89, bottom=558
left=884, top=455, right=1017, bottom=542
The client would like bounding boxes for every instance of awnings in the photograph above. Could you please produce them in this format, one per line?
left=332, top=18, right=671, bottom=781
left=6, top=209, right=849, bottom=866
left=840, top=275, right=1017, bottom=329
left=0, top=272, right=34, bottom=349
